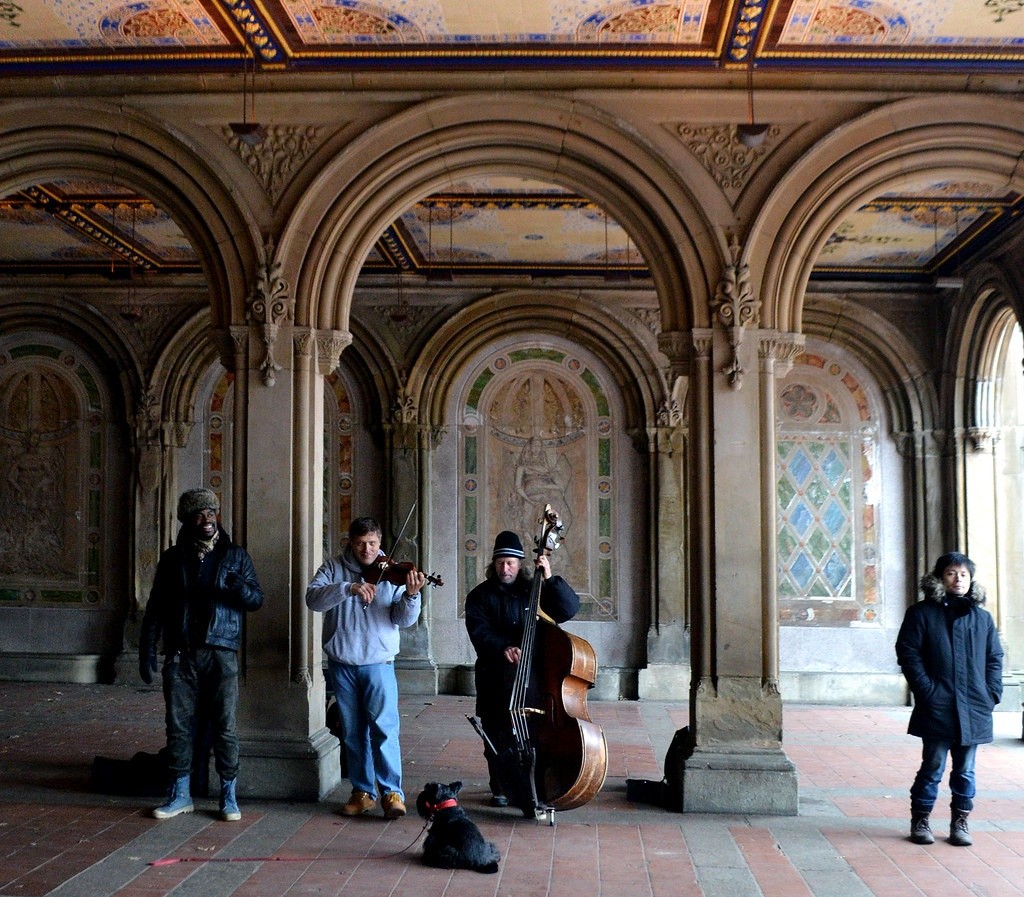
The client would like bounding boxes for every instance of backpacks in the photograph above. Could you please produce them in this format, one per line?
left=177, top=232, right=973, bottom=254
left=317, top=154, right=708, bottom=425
left=661, top=726, right=690, bottom=784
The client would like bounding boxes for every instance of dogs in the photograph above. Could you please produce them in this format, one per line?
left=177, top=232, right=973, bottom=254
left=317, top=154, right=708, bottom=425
left=416, top=781, right=500, bottom=869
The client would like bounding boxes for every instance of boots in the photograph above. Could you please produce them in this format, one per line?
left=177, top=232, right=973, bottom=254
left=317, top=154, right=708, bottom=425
left=152, top=775, right=195, bottom=818
left=219, top=777, right=242, bottom=820
left=950, top=808, right=973, bottom=844
left=910, top=808, right=935, bottom=842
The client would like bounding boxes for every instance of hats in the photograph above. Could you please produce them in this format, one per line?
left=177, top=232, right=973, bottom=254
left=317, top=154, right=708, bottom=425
left=492, top=531, right=525, bottom=559
left=177, top=487, right=220, bottom=523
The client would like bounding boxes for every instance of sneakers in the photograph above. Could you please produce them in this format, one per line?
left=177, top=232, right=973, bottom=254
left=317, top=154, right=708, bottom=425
left=342, top=791, right=376, bottom=814
left=381, top=795, right=407, bottom=817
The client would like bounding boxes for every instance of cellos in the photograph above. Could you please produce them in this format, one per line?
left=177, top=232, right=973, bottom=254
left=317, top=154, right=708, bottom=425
left=484, top=502, right=609, bottom=817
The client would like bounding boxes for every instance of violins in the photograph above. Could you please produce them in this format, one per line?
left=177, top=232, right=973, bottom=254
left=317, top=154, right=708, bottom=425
left=363, top=557, right=445, bottom=590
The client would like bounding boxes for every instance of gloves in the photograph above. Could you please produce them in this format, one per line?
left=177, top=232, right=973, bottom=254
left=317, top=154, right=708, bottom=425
left=224, top=573, right=243, bottom=592
left=138, top=645, right=158, bottom=684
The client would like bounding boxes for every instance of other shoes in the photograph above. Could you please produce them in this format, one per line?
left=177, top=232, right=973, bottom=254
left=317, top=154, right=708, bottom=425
left=491, top=790, right=510, bottom=806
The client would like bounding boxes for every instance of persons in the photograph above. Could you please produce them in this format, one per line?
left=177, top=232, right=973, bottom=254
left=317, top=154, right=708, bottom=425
left=138, top=486, right=266, bottom=821
left=306, top=517, right=426, bottom=820
left=894, top=552, right=1003, bottom=845
left=465, top=531, right=583, bottom=820
